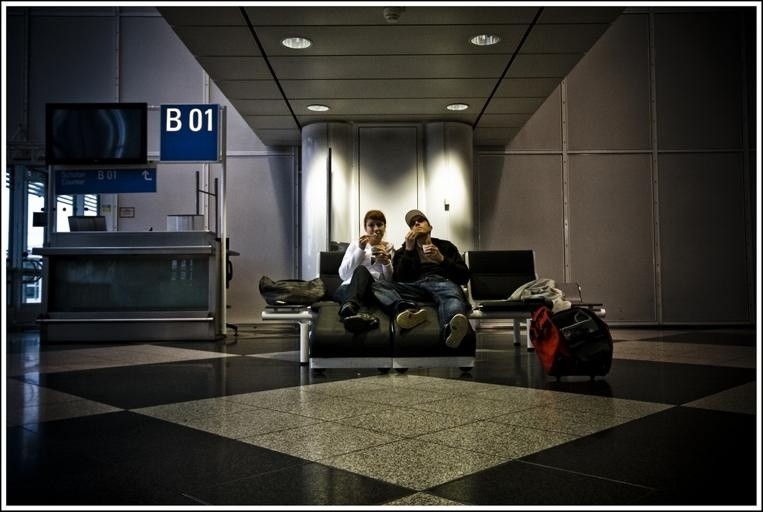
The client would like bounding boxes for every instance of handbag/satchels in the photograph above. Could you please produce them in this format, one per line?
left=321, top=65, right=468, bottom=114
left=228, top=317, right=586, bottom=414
left=529, top=306, right=613, bottom=382
left=259, top=275, right=327, bottom=305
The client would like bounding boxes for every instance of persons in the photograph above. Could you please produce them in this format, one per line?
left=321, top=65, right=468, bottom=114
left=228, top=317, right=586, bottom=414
left=391, top=209, right=472, bottom=348
left=332, top=210, right=394, bottom=334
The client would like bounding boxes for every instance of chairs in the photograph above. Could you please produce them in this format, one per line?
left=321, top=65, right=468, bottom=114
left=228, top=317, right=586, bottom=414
left=226, top=237, right=240, bottom=336
left=311, top=250, right=603, bottom=375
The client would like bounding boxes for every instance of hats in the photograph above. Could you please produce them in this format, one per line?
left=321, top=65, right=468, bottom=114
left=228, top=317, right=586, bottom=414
left=405, top=209, right=430, bottom=228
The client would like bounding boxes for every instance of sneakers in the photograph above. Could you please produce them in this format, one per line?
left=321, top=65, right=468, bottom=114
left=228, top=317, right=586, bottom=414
left=444, top=313, right=470, bottom=349
left=339, top=302, right=379, bottom=332
left=396, top=307, right=428, bottom=329
